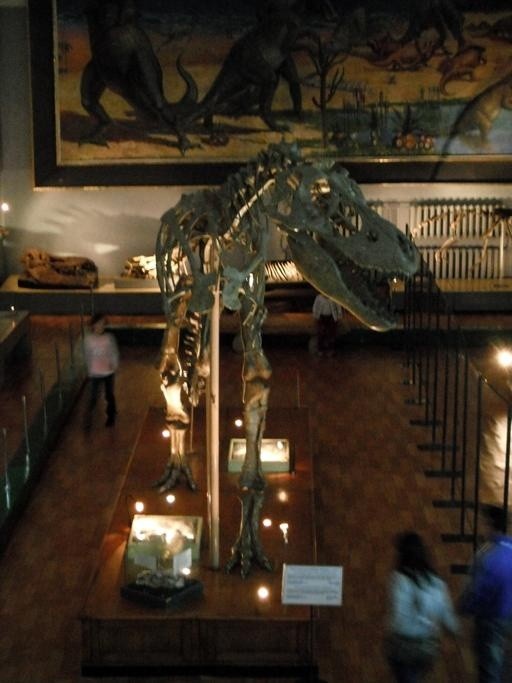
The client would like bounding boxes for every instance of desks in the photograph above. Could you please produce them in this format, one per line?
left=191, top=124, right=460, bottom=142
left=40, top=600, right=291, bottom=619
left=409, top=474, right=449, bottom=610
left=77, top=407, right=329, bottom=683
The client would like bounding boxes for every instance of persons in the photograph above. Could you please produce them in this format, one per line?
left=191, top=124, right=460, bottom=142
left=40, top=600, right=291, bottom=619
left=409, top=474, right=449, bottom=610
left=389, top=531, right=477, bottom=683
left=454, top=503, right=512, bottom=683
left=313, top=292, right=343, bottom=357
left=80, top=315, right=121, bottom=433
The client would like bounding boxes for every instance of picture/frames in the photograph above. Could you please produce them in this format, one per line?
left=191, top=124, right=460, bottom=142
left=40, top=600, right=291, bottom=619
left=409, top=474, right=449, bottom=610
left=26, top=1, right=510, bottom=191
